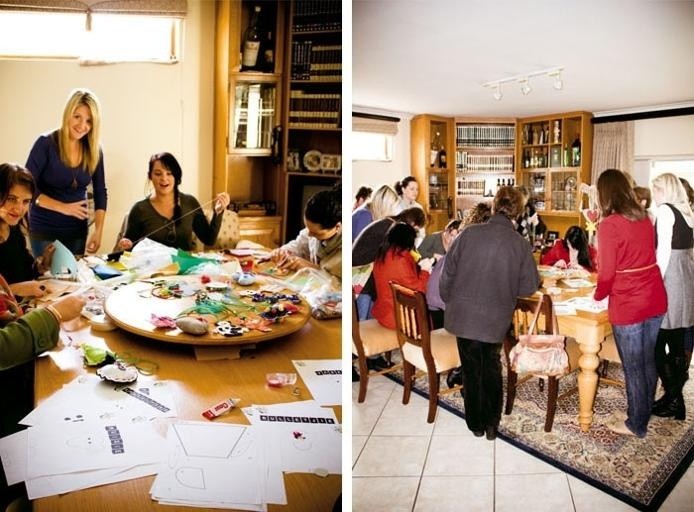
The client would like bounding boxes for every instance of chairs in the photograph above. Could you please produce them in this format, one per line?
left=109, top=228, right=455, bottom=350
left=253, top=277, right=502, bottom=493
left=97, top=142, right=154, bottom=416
left=387, top=280, right=462, bottom=424
left=352, top=286, right=415, bottom=402
left=503, top=292, right=583, bottom=433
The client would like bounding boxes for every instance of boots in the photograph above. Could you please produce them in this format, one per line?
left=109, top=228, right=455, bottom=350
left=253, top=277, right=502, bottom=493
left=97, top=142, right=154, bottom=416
left=653, top=362, right=686, bottom=420
left=650, top=355, right=692, bottom=413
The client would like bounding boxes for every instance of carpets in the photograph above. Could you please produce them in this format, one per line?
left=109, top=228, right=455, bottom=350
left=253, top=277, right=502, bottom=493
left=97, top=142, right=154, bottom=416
left=352, top=348, right=694, bottom=511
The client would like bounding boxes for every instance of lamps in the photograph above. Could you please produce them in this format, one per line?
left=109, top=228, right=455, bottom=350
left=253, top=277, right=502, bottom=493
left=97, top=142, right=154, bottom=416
left=492, top=66, right=565, bottom=103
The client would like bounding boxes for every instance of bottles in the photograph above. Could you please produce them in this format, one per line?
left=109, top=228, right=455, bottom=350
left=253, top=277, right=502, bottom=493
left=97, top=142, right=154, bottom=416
left=237, top=5, right=274, bottom=73
left=495, top=177, right=515, bottom=192
left=523, top=121, right=581, bottom=168
left=432, top=132, right=447, bottom=168
left=429, top=183, right=448, bottom=209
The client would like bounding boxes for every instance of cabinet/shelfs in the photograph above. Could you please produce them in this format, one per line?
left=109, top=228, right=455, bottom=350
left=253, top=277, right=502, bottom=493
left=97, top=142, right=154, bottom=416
left=410, top=111, right=595, bottom=238
left=210, top=1, right=284, bottom=250
left=279, top=0, right=342, bottom=247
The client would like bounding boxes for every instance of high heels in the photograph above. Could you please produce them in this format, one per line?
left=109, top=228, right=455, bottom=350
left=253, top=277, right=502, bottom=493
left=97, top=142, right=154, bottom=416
left=446, top=369, right=466, bottom=389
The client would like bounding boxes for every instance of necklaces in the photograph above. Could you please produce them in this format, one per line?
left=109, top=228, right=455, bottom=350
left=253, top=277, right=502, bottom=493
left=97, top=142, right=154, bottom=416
left=71, top=167, right=80, bottom=193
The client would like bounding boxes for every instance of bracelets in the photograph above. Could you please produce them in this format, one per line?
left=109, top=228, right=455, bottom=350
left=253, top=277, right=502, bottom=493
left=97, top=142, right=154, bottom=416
left=48, top=304, right=62, bottom=322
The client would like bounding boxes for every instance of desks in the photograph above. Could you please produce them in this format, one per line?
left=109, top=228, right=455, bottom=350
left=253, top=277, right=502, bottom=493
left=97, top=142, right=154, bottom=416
left=510, top=263, right=613, bottom=433
left=29, top=249, right=342, bottom=510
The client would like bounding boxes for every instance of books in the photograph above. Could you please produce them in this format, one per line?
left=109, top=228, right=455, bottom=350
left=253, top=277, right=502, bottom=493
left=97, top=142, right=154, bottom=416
left=289, top=1, right=342, bottom=131
left=456, top=123, right=514, bottom=198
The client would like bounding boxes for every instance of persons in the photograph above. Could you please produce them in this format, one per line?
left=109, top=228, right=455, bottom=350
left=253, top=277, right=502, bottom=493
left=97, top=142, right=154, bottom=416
left=24, top=90, right=107, bottom=263
left=0, top=275, right=87, bottom=371
left=272, top=185, right=342, bottom=282
left=0, top=162, right=55, bottom=283
left=118, top=152, right=231, bottom=252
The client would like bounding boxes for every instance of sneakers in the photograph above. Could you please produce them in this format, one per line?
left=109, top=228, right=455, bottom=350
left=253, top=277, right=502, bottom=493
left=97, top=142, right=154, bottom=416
left=625, top=419, right=647, bottom=438
left=470, top=426, right=483, bottom=437
left=485, top=425, right=499, bottom=441
left=352, top=366, right=361, bottom=383
left=361, top=352, right=396, bottom=371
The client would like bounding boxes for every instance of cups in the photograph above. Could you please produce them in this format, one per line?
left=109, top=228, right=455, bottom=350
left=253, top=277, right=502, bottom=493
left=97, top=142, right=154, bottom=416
left=529, top=173, right=577, bottom=212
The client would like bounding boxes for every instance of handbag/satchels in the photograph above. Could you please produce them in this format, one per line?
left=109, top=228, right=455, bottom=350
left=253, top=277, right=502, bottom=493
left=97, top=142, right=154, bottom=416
left=509, top=293, right=572, bottom=379
